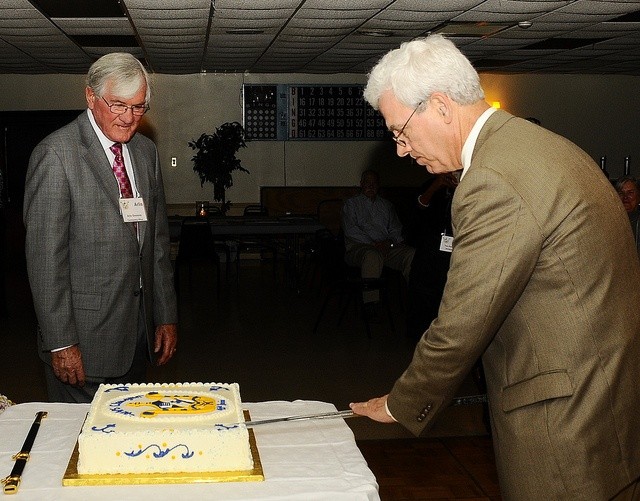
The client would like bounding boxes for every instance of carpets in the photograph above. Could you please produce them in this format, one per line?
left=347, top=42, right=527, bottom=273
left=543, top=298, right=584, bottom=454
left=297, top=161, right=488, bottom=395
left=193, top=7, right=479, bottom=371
left=359, top=437, right=504, bottom=501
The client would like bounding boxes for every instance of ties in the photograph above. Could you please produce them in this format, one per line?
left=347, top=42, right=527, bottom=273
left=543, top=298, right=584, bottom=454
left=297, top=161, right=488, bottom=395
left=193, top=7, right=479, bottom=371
left=110, top=142, right=138, bottom=235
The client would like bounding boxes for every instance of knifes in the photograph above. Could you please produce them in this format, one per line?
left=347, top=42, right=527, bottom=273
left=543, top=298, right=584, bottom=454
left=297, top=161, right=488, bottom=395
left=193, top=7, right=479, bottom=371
left=245, top=393, right=487, bottom=428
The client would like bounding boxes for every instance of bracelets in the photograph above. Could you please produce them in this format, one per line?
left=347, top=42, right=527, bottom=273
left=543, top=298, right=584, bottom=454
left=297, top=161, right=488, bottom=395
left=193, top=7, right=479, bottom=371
left=417, top=194, right=429, bottom=207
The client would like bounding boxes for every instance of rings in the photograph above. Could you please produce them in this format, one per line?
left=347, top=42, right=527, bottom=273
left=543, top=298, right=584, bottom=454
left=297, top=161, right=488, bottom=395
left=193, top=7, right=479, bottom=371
left=170, top=348, right=177, bottom=353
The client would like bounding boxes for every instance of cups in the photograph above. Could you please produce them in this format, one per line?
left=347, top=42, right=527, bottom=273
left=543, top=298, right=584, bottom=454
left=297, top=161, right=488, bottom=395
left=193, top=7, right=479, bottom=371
left=195, top=200, right=210, bottom=222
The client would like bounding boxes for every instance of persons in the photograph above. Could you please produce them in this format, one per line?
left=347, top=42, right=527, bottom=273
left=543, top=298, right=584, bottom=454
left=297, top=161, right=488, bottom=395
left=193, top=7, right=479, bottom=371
left=348, top=34, right=640, bottom=501
left=614, top=175, right=639, bottom=260
left=340, top=169, right=414, bottom=312
left=402, top=169, right=463, bottom=335
left=23, top=51, right=178, bottom=402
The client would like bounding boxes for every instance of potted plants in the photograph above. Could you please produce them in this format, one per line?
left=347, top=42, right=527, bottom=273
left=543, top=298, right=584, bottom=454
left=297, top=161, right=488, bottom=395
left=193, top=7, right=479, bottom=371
left=188, top=122, right=250, bottom=214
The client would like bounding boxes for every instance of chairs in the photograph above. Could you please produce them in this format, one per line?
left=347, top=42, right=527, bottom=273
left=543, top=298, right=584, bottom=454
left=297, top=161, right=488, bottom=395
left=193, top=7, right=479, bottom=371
left=312, top=228, right=396, bottom=338
left=176, top=215, right=220, bottom=300
left=238, top=202, right=273, bottom=281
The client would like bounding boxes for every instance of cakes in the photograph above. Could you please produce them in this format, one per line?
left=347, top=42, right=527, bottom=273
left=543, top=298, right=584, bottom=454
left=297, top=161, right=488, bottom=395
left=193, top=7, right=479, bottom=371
left=76, top=381, right=253, bottom=475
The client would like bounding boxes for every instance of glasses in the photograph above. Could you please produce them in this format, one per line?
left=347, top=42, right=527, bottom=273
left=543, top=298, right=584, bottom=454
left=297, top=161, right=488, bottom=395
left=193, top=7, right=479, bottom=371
left=101, top=96, right=151, bottom=115
left=392, top=101, right=424, bottom=147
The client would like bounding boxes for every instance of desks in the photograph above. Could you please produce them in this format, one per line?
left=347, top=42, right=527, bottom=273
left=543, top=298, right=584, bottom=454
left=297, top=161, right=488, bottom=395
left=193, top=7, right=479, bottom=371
left=208, top=215, right=328, bottom=313
